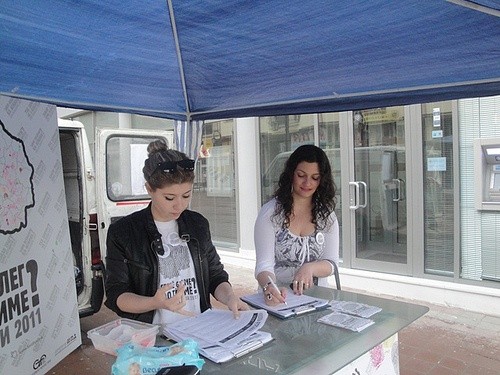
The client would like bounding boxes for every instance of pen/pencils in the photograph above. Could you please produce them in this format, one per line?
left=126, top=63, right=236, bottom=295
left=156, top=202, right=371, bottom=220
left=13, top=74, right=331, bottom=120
left=268, top=275, right=288, bottom=307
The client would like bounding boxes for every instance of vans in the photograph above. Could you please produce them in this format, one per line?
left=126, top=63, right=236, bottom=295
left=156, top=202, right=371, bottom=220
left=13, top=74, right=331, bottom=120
left=56, top=118, right=175, bottom=318
left=262, top=146, right=406, bottom=230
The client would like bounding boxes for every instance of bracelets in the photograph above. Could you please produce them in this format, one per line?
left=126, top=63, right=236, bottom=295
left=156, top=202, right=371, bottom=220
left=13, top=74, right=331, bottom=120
left=263, top=282, right=271, bottom=291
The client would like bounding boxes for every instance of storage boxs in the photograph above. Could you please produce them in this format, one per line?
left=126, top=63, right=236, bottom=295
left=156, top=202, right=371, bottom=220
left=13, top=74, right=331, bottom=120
left=87, top=319, right=161, bottom=357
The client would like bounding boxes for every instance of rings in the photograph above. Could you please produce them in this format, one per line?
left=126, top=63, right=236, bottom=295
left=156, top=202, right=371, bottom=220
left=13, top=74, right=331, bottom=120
left=266, top=294, right=272, bottom=300
left=293, top=280, right=297, bottom=283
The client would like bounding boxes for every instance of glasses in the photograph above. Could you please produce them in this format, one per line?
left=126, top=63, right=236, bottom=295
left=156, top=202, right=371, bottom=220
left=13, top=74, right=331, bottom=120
left=149, top=159, right=195, bottom=180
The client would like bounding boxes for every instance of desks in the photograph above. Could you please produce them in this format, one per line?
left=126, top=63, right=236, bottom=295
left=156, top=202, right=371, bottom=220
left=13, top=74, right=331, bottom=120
left=114, top=279, right=429, bottom=375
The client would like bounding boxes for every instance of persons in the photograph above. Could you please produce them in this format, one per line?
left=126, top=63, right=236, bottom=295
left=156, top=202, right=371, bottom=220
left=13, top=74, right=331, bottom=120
left=254, top=145, right=340, bottom=307
left=104, top=140, right=249, bottom=324
